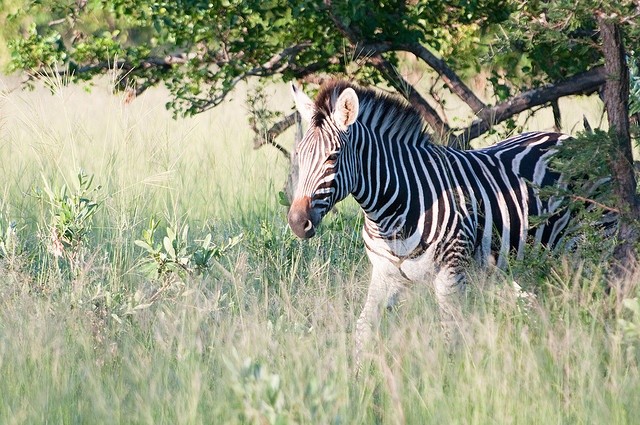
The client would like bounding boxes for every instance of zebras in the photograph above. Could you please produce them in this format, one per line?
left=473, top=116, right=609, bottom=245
left=285, top=73, right=622, bottom=381
